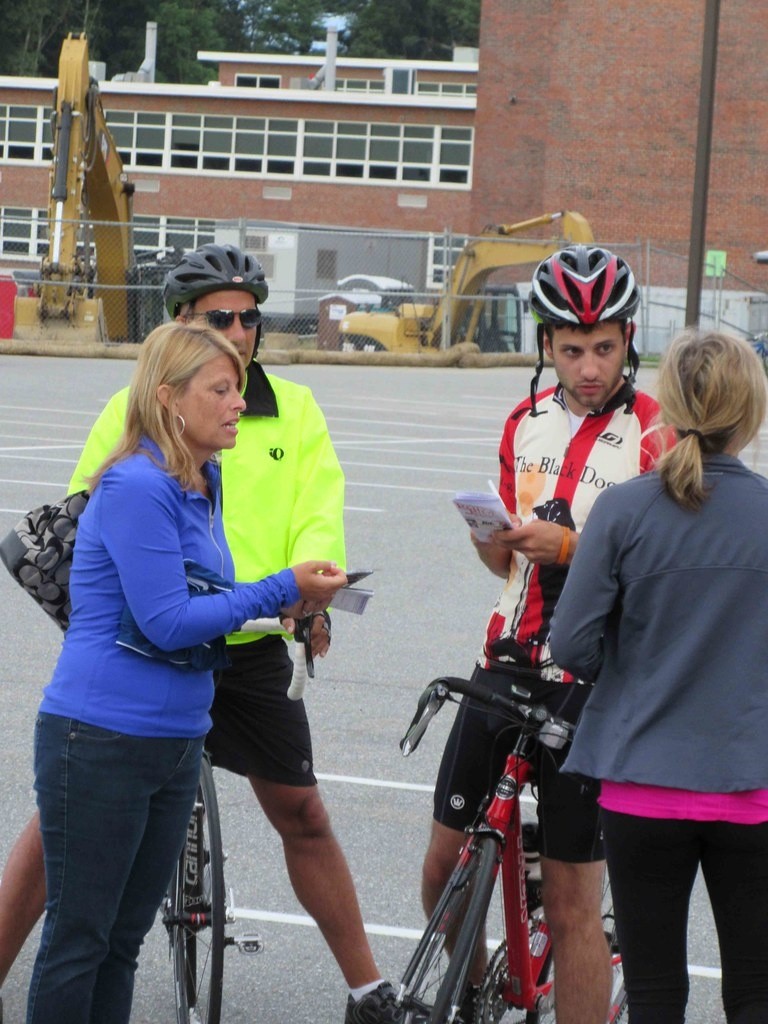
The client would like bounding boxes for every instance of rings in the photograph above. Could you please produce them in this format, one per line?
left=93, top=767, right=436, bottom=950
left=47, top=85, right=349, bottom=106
left=302, top=607, right=313, bottom=617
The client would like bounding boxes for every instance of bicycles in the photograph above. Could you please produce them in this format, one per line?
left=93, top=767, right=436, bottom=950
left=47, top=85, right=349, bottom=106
left=390, top=637, right=631, bottom=1024
left=152, top=587, right=317, bottom=1024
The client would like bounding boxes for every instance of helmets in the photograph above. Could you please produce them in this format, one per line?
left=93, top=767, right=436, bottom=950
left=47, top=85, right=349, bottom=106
left=161, top=243, right=268, bottom=321
left=527, top=243, right=640, bottom=324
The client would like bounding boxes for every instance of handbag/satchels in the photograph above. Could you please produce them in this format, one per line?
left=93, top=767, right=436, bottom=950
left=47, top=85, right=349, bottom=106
left=115, top=556, right=236, bottom=671
left=0, top=489, right=94, bottom=636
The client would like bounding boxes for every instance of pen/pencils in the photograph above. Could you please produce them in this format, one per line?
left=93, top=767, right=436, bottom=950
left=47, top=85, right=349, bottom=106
left=488, top=479, right=508, bottom=512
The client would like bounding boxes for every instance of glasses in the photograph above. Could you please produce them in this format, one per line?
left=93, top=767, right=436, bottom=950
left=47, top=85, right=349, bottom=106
left=183, top=309, right=262, bottom=330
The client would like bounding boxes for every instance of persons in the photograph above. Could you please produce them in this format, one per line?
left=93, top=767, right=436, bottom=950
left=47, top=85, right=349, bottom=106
left=549, top=329, right=768, bottom=1024
left=0, top=244, right=459, bottom=1024
left=420, top=246, right=678, bottom=1024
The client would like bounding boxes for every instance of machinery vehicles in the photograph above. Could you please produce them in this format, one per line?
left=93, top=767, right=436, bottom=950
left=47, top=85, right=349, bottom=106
left=14, top=30, right=171, bottom=343
left=337, top=208, right=595, bottom=355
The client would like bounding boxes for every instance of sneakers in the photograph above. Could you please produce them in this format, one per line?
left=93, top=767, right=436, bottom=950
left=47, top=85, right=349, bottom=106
left=343, top=982, right=433, bottom=1024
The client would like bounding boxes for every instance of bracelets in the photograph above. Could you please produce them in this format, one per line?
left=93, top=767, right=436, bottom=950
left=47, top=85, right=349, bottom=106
left=556, top=527, right=570, bottom=565
